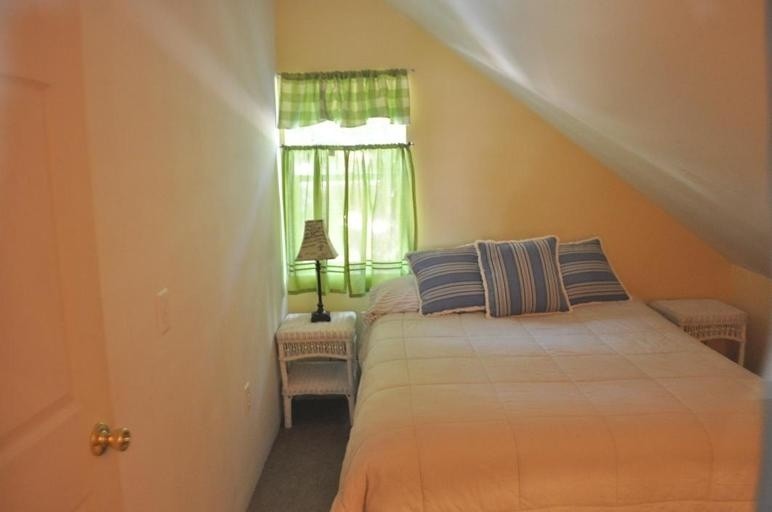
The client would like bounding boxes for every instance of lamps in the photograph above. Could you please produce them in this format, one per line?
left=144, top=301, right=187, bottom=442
left=295, top=220, right=338, bottom=322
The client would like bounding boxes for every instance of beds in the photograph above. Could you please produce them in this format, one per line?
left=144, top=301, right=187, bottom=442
left=330, top=298, right=765, bottom=512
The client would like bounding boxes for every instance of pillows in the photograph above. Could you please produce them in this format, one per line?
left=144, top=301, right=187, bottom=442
left=559, top=236, right=632, bottom=306
left=403, top=239, right=485, bottom=316
left=475, top=234, right=573, bottom=318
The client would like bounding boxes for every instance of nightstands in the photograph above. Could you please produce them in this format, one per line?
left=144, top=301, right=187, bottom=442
left=275, top=312, right=358, bottom=429
left=648, top=299, right=749, bottom=365
